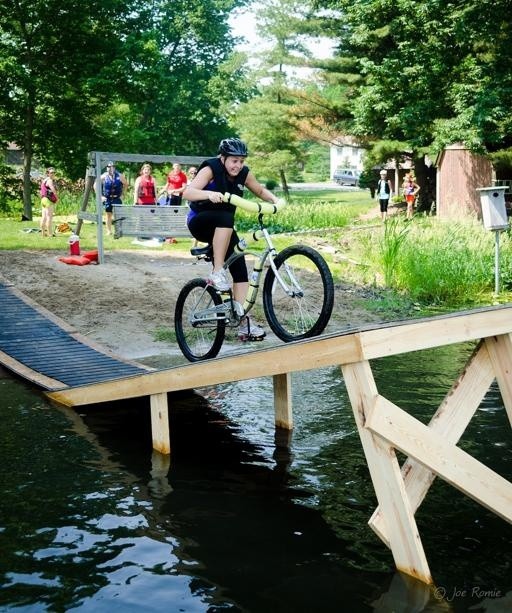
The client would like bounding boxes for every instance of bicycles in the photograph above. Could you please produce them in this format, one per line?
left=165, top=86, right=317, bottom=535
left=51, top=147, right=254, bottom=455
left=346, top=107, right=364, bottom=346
left=174, top=192, right=334, bottom=362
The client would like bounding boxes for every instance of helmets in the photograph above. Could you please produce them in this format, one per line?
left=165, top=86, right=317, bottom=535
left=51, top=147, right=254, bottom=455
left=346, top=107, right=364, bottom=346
left=380, top=169, right=387, bottom=175
left=218, top=137, right=248, bottom=158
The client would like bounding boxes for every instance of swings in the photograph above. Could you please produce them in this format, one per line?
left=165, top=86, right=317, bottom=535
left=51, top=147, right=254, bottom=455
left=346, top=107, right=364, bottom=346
left=109, top=161, right=195, bottom=238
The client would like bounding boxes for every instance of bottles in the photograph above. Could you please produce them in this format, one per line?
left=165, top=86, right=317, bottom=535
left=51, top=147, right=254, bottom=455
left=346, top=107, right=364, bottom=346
left=234, top=239, right=246, bottom=254
left=70, top=235, right=80, bottom=255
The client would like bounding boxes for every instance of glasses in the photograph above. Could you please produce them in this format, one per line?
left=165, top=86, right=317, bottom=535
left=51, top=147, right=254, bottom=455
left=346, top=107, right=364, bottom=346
left=51, top=172, right=56, bottom=174
left=107, top=165, right=116, bottom=168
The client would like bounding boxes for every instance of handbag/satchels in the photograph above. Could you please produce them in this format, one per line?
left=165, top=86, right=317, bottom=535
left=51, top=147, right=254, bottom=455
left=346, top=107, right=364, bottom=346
left=44, top=178, right=57, bottom=203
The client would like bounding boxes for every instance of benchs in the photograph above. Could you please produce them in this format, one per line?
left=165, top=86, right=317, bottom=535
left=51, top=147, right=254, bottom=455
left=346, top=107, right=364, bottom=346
left=111, top=204, right=192, bottom=240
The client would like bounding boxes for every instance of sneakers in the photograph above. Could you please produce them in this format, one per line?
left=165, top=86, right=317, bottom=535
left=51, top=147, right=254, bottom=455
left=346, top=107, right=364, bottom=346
left=205, top=268, right=232, bottom=292
left=237, top=326, right=266, bottom=338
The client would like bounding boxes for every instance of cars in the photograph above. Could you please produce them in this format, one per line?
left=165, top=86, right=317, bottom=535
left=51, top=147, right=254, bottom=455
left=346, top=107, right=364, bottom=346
left=332, top=168, right=362, bottom=186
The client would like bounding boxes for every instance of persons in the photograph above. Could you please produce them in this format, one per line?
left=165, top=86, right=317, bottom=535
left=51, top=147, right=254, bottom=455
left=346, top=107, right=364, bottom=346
left=40, top=166, right=61, bottom=238
left=93, top=160, right=129, bottom=236
left=133, top=163, right=159, bottom=241
left=182, top=135, right=287, bottom=342
left=374, top=168, right=393, bottom=223
left=158, top=161, right=188, bottom=242
left=183, top=165, right=199, bottom=206
left=400, top=172, right=421, bottom=221
